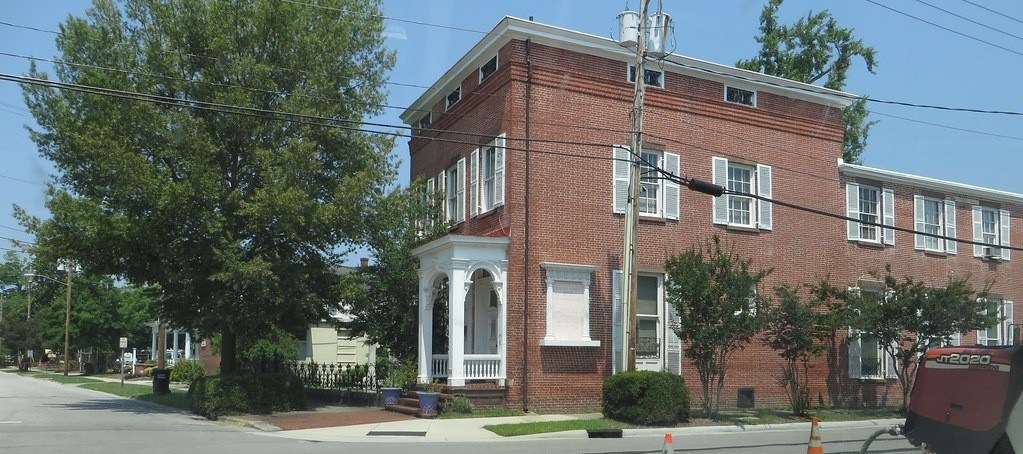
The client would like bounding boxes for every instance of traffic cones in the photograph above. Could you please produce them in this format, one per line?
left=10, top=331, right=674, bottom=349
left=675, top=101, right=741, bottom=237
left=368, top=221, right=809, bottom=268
left=661, top=433, right=677, bottom=454
left=806, top=417, right=824, bottom=454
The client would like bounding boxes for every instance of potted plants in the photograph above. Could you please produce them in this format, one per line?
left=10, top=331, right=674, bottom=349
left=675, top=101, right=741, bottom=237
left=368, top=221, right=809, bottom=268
left=416, top=378, right=446, bottom=410
left=380, top=369, right=406, bottom=405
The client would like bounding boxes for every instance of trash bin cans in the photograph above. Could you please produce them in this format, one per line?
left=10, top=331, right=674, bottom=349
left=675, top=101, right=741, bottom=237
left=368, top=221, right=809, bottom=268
left=151, top=368, right=173, bottom=395
left=737, top=386, right=755, bottom=408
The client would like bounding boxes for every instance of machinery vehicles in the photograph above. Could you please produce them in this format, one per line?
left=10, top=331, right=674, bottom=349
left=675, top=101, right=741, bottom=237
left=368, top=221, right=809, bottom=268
left=902, top=323, right=1023, bottom=454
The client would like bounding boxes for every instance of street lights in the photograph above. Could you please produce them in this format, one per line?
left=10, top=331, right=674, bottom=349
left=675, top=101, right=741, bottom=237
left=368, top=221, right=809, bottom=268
left=23, top=273, right=71, bottom=375
